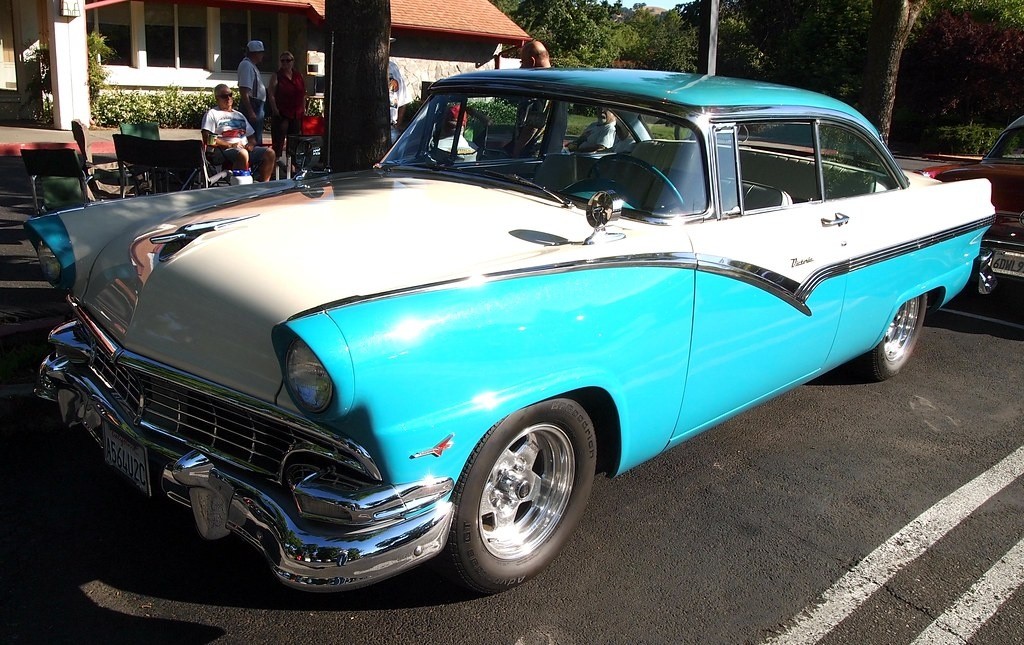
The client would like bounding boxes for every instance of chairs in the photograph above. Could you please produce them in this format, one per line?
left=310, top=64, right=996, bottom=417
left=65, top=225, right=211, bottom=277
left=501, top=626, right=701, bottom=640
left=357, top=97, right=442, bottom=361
left=20, top=118, right=276, bottom=214
left=642, top=165, right=793, bottom=218
left=534, top=153, right=599, bottom=191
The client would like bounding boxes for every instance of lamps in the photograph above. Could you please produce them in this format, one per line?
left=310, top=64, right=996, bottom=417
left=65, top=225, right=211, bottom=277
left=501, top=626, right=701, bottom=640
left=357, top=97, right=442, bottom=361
left=59, top=0, right=81, bottom=17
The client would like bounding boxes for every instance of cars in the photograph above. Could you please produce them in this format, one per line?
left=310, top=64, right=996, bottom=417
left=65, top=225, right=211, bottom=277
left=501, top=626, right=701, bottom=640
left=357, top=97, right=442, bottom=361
left=24, top=68, right=996, bottom=595
left=913, top=115, right=1024, bottom=282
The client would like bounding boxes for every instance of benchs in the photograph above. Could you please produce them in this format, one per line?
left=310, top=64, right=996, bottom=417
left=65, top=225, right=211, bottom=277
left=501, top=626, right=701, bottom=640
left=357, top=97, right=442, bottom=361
left=620, top=139, right=883, bottom=200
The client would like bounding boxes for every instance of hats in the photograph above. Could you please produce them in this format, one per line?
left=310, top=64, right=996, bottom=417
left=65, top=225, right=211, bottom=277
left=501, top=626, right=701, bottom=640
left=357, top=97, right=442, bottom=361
left=247, top=41, right=264, bottom=53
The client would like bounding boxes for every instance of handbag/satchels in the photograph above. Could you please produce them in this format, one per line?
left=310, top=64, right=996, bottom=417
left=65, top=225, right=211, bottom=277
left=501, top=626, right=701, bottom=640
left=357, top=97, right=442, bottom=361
left=263, top=72, right=279, bottom=117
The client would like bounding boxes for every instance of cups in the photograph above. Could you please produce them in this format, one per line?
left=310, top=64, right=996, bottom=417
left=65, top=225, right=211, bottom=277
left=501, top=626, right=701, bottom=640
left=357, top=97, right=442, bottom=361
left=206, top=132, right=218, bottom=152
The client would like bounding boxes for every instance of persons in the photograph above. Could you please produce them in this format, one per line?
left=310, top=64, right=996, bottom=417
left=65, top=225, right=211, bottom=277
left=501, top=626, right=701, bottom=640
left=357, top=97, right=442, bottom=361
left=203, top=81, right=275, bottom=184
left=566, top=105, right=617, bottom=152
left=237, top=41, right=267, bottom=138
left=501, top=41, right=552, bottom=159
left=268, top=51, right=307, bottom=178
left=435, top=103, right=478, bottom=163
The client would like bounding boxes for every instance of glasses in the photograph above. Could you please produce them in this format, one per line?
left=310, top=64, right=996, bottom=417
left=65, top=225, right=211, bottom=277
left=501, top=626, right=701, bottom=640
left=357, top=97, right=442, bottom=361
left=281, top=59, right=292, bottom=63
left=216, top=92, right=233, bottom=99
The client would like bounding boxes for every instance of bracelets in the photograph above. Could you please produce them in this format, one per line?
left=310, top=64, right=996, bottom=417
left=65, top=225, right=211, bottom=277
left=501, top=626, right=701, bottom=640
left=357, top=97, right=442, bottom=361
left=247, top=109, right=254, bottom=115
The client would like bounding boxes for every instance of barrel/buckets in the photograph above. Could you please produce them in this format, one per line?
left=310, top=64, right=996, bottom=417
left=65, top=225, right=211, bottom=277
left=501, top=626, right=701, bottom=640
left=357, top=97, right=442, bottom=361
left=230, top=169, right=254, bottom=187
